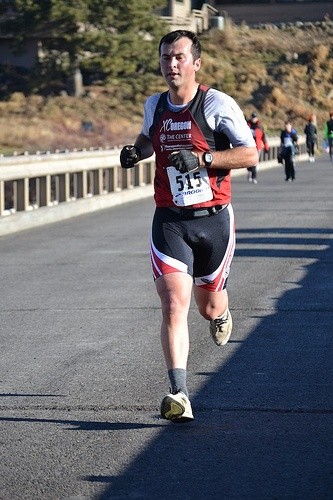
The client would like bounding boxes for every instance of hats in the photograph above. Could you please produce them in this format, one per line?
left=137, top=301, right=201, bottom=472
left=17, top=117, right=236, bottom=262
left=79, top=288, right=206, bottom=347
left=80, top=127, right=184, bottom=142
left=251, top=113, right=258, bottom=118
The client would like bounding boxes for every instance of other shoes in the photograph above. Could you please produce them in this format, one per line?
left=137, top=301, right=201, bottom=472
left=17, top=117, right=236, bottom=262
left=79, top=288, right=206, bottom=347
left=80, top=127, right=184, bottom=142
left=308, top=156, right=315, bottom=163
left=253, top=178, right=258, bottom=183
left=285, top=176, right=296, bottom=181
left=247, top=175, right=252, bottom=183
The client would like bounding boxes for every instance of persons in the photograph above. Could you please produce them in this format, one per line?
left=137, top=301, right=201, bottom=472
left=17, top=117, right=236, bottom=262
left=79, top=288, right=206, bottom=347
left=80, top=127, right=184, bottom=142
left=119, top=30, right=259, bottom=424
left=323, top=112, right=333, bottom=162
left=277, top=119, right=299, bottom=181
left=304, top=114, right=320, bottom=162
left=244, top=112, right=269, bottom=183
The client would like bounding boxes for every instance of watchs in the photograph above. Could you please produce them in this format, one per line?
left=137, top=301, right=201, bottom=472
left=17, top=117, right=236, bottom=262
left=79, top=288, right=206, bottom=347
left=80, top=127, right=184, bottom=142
left=203, top=151, right=212, bottom=167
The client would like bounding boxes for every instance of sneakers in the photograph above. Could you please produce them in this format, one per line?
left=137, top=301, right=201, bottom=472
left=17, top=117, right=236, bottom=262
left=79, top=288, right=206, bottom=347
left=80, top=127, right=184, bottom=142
left=160, top=391, right=194, bottom=423
left=210, top=306, right=233, bottom=346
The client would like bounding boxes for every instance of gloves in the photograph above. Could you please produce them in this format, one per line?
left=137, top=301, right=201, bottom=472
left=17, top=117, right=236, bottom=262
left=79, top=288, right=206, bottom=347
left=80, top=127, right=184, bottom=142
left=167, top=150, right=201, bottom=174
left=120, top=145, right=142, bottom=169
